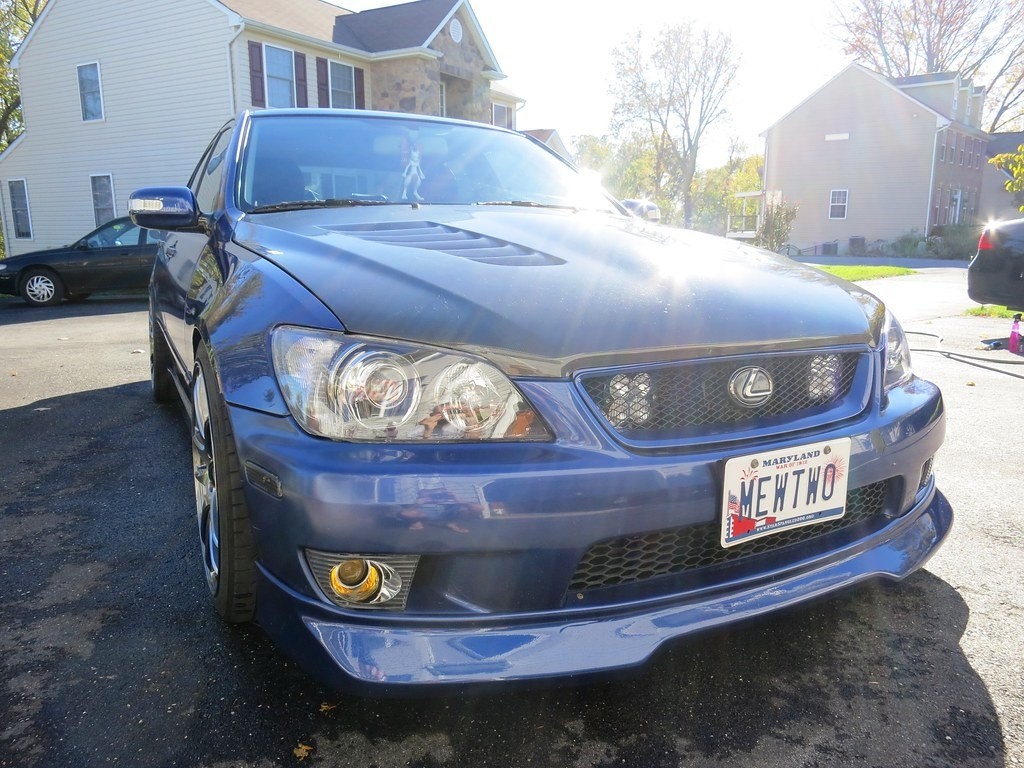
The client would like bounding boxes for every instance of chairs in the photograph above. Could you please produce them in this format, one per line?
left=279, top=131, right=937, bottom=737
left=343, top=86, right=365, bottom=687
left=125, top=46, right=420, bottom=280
left=252, top=157, right=318, bottom=207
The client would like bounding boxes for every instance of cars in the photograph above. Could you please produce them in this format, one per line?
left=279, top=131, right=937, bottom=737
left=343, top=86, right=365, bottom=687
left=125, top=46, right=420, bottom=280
left=126, top=106, right=954, bottom=698
left=966, top=219, right=1024, bottom=314
left=0, top=216, right=167, bottom=309
